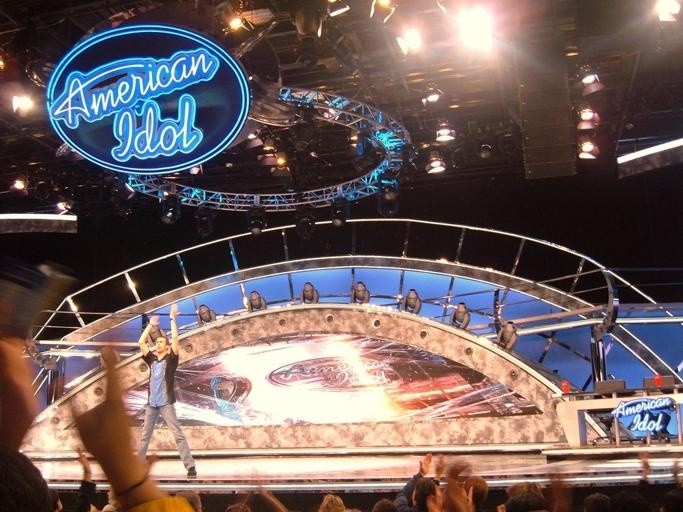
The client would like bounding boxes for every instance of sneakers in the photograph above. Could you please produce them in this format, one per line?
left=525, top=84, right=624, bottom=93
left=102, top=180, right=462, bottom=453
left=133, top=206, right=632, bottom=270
left=187, top=467, right=197, bottom=478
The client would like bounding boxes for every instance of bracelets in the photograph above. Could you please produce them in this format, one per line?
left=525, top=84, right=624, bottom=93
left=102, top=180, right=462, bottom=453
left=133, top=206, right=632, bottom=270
left=170, top=318, right=175, bottom=321
left=149, top=323, right=153, bottom=327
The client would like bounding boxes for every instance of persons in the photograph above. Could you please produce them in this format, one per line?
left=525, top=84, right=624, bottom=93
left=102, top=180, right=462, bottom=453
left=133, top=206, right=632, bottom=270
left=49, top=447, right=683, bottom=512
left=137, top=302, right=198, bottom=478
left=0, top=337, right=197, bottom=512
